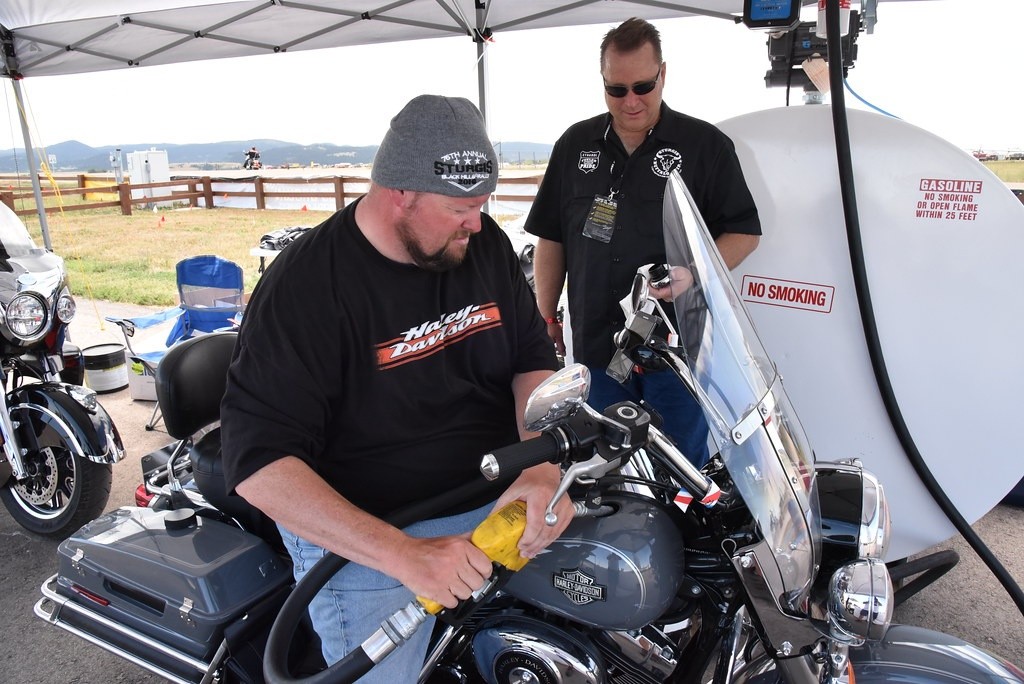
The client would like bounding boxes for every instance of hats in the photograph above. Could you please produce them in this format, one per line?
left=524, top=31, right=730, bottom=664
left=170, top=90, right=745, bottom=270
left=371, top=95, right=499, bottom=197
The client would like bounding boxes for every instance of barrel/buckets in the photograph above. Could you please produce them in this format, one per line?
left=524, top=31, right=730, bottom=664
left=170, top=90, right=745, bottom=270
left=82, top=343, right=129, bottom=393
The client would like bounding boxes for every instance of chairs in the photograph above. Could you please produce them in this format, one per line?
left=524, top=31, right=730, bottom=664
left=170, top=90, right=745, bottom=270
left=104, top=253, right=247, bottom=431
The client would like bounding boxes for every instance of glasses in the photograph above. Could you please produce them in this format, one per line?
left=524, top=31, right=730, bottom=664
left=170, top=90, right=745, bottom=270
left=603, top=68, right=660, bottom=98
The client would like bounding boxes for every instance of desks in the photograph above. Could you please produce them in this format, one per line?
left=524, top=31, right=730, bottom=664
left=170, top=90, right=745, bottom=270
left=249, top=248, right=283, bottom=273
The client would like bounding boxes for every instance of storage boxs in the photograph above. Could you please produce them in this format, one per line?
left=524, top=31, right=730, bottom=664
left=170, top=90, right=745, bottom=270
left=59, top=341, right=84, bottom=386
left=52, top=506, right=289, bottom=684
left=126, top=351, right=159, bottom=401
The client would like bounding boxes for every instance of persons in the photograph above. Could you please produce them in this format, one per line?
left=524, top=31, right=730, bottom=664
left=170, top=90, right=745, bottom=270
left=523, top=16, right=764, bottom=373
left=220, top=95, right=577, bottom=684
left=243, top=145, right=259, bottom=168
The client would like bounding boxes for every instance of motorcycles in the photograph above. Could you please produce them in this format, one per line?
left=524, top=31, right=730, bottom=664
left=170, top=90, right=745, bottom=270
left=33, top=165, right=1023, bottom=683
left=243, top=151, right=263, bottom=171
left=1, top=200, right=128, bottom=544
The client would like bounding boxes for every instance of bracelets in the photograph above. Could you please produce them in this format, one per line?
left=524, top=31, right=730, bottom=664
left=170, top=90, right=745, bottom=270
left=544, top=317, right=558, bottom=324
